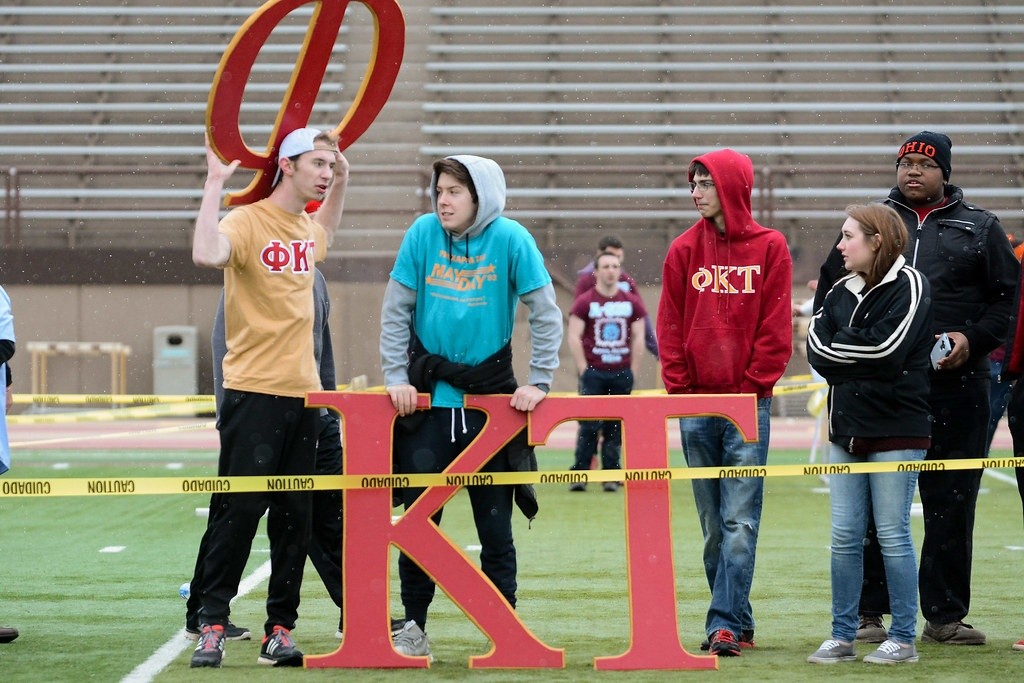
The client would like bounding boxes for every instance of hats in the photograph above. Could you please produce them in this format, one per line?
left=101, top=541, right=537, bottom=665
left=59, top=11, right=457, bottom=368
left=896, top=131, right=952, bottom=182
left=271, top=128, right=338, bottom=187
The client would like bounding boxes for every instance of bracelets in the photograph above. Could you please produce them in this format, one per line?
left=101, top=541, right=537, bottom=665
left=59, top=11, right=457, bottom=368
left=535, top=383, right=549, bottom=394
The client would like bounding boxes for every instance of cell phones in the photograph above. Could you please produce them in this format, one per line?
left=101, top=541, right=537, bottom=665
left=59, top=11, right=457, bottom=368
left=930, top=333, right=952, bottom=370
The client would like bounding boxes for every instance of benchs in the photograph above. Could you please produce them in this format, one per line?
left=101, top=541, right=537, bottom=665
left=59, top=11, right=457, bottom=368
left=0, top=0, right=1024, bottom=254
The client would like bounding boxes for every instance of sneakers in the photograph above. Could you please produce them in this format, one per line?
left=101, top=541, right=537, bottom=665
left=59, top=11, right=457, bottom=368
left=856, top=615, right=888, bottom=642
left=392, top=620, right=428, bottom=656
left=807, top=641, right=856, bottom=663
left=708, top=630, right=742, bottom=655
left=922, top=620, right=987, bottom=645
left=701, top=632, right=754, bottom=650
left=257, top=625, right=304, bottom=666
left=190, top=623, right=225, bottom=667
left=863, top=640, right=918, bottom=664
left=185, top=621, right=251, bottom=641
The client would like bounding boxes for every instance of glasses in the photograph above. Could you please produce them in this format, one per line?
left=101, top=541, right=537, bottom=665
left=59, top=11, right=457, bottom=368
left=897, top=163, right=940, bottom=172
left=689, top=183, right=714, bottom=192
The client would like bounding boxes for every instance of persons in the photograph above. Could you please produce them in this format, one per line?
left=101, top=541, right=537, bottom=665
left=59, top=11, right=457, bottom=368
left=656, top=147, right=794, bottom=656
left=0, top=285, right=20, bottom=645
left=570, top=234, right=659, bottom=493
left=1005, top=231, right=1024, bottom=652
left=182, top=199, right=399, bottom=640
left=376, top=153, right=563, bottom=657
left=809, top=204, right=934, bottom=668
left=187, top=127, right=349, bottom=669
left=814, top=130, right=1024, bottom=646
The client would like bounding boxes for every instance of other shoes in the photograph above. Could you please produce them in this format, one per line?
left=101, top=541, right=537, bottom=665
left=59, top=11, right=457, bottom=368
left=571, top=483, right=585, bottom=491
left=0, top=627, right=18, bottom=642
left=1012, top=639, right=1024, bottom=649
left=604, top=482, right=617, bottom=491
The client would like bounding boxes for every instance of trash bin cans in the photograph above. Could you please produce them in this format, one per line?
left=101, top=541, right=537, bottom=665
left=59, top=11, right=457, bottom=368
left=152, top=326, right=198, bottom=396
